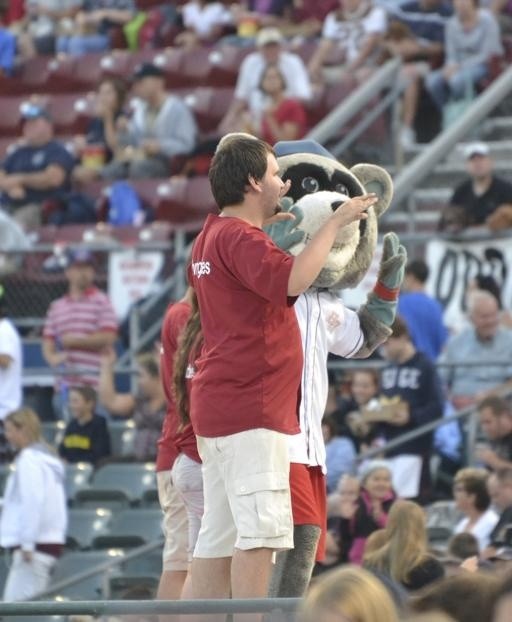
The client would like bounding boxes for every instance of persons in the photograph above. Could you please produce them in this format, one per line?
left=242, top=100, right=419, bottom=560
left=1, top=0, right=511, bottom=621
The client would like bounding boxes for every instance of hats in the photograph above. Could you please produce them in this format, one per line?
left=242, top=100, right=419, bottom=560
left=463, top=144, right=488, bottom=159
left=255, top=31, right=283, bottom=52
left=131, top=64, right=168, bottom=83
left=21, top=100, right=51, bottom=120
